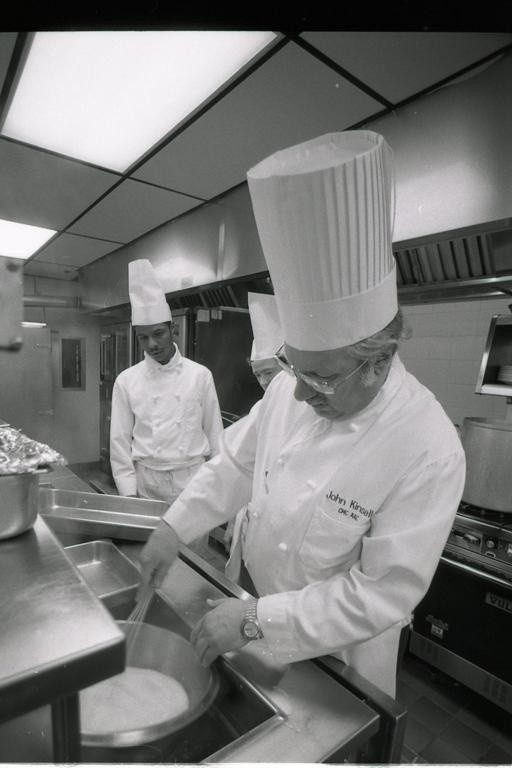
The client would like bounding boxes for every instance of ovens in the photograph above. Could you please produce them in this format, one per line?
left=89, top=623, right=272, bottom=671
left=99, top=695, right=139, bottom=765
left=405, top=549, right=512, bottom=713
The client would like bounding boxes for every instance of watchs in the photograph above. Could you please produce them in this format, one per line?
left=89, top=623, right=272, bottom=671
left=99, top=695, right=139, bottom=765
left=240, top=596, right=266, bottom=641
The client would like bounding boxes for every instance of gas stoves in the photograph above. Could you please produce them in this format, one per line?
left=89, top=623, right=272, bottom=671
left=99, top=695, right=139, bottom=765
left=444, top=501, right=512, bottom=581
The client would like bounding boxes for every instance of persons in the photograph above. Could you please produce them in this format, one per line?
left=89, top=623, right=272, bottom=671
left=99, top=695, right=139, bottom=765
left=109, top=312, right=223, bottom=501
left=246, top=350, right=286, bottom=390
left=133, top=305, right=467, bottom=698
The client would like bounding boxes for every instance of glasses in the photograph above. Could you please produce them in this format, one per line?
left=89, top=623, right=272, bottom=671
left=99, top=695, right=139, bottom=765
left=273, top=342, right=368, bottom=395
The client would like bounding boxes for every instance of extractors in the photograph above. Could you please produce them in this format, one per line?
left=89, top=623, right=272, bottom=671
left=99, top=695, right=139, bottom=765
left=475, top=314, right=512, bottom=403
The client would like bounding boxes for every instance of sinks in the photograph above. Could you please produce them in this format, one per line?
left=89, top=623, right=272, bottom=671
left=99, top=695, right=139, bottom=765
left=78, top=586, right=285, bottom=764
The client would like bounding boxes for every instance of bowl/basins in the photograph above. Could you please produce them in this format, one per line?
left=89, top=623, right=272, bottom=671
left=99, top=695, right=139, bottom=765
left=78, top=618, right=218, bottom=762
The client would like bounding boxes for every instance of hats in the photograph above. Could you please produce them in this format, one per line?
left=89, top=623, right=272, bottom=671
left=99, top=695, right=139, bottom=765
left=128, top=259, right=172, bottom=326
left=246, top=130, right=398, bottom=351
left=248, top=292, right=283, bottom=361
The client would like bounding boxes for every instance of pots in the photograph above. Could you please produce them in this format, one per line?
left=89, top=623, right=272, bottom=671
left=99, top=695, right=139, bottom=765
left=461, top=416, right=511, bottom=513
